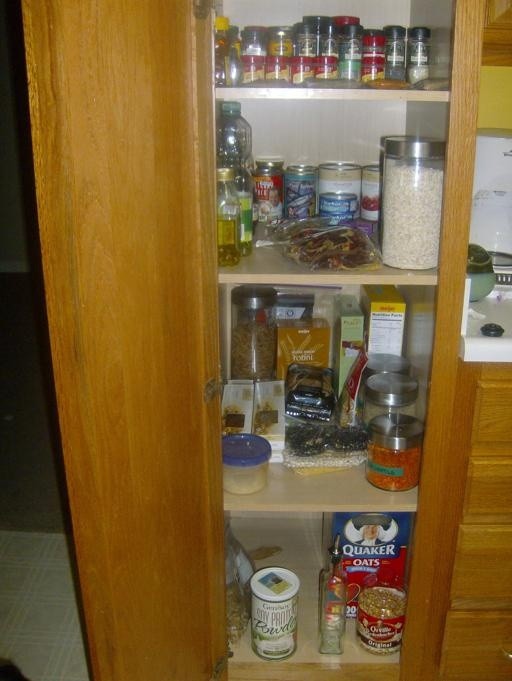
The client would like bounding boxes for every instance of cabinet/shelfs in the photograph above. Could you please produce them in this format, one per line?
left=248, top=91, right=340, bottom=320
left=17, top=2, right=485, bottom=679
left=435, top=358, right=512, bottom=681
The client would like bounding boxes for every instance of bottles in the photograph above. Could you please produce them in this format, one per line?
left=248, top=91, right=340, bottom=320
left=267, top=25, right=294, bottom=56
left=315, top=56, right=337, bottom=83
left=317, top=533, right=346, bottom=656
left=240, top=24, right=266, bottom=55
left=251, top=158, right=285, bottom=225
left=223, top=511, right=256, bottom=635
left=363, top=28, right=385, bottom=82
left=295, top=23, right=317, bottom=57
left=216, top=167, right=242, bottom=265
left=265, top=55, right=289, bottom=84
left=431, top=26, right=451, bottom=79
left=319, top=22, right=338, bottom=56
left=231, top=284, right=277, bottom=379
left=229, top=23, right=241, bottom=86
left=214, top=16, right=230, bottom=86
left=241, top=56, right=264, bottom=85
left=367, top=353, right=410, bottom=380
left=215, top=101, right=254, bottom=256
left=384, top=25, right=408, bottom=81
left=291, top=56, right=314, bottom=84
left=339, top=23, right=362, bottom=81
left=407, top=25, right=430, bottom=83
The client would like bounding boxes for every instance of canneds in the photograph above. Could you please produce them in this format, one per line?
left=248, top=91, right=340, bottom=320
left=360, top=163, right=382, bottom=215
left=318, top=163, right=360, bottom=197
left=317, top=195, right=359, bottom=227
left=359, top=213, right=380, bottom=241
left=255, top=158, right=281, bottom=223
left=366, top=352, right=411, bottom=384
left=249, top=568, right=301, bottom=661
left=281, top=165, right=317, bottom=222
